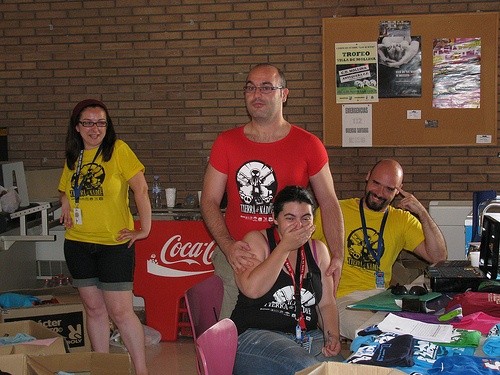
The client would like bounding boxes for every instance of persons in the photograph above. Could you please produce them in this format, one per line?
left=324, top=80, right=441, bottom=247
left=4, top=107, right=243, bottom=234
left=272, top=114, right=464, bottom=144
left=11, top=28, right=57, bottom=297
left=200, top=64, right=345, bottom=320
left=230, top=186, right=345, bottom=375
left=312, top=159, right=448, bottom=341
left=58, top=99, right=151, bottom=375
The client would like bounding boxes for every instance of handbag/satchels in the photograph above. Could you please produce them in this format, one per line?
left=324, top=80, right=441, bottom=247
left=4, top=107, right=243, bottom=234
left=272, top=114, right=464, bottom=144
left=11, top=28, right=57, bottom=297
left=348, top=334, right=415, bottom=367
left=445, top=292, right=500, bottom=318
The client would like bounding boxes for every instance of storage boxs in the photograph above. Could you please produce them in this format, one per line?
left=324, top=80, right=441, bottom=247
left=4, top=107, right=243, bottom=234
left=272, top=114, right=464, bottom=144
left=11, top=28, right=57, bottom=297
left=0, top=291, right=132, bottom=375
left=428, top=201, right=474, bottom=261
left=296, top=361, right=405, bottom=375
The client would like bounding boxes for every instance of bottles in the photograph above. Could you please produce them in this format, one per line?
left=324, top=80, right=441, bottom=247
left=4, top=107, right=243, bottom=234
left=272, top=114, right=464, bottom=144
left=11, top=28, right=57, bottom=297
left=151, top=176, right=162, bottom=209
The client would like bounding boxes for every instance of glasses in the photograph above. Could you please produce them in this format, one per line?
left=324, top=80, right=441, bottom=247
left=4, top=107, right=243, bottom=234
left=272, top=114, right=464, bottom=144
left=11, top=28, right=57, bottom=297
left=391, top=282, right=429, bottom=295
left=78, top=120, right=109, bottom=128
left=243, top=86, right=284, bottom=94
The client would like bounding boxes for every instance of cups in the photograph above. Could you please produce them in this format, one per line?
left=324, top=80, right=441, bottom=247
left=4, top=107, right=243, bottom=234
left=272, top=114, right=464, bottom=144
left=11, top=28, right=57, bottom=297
left=198, top=191, right=201, bottom=202
left=165, top=188, right=176, bottom=207
left=470, top=252, right=480, bottom=267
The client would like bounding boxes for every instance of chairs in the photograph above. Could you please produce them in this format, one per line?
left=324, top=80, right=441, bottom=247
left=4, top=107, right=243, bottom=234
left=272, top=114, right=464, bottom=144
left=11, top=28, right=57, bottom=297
left=185, top=275, right=223, bottom=341
left=196, top=318, right=238, bottom=375
